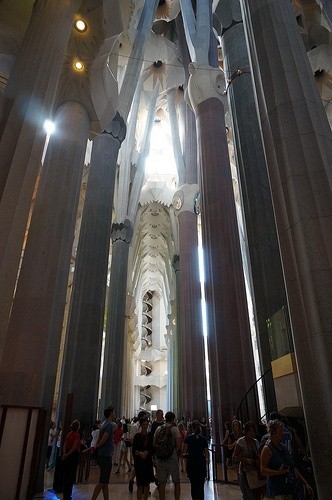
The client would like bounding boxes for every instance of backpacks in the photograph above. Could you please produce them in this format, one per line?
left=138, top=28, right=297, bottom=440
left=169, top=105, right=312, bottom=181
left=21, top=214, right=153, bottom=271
left=154, top=423, right=177, bottom=459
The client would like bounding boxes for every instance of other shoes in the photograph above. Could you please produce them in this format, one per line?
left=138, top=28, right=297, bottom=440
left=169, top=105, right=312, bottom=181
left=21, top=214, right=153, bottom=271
left=128, top=480, right=134, bottom=493
left=113, top=462, right=118, bottom=466
left=46, top=468, right=53, bottom=472
left=154, top=475, right=159, bottom=486
left=126, top=468, right=132, bottom=473
left=113, top=469, right=120, bottom=474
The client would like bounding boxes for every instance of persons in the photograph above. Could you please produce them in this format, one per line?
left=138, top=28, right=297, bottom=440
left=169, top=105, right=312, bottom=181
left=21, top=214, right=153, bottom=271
left=61, top=420, right=81, bottom=500
left=232, top=422, right=263, bottom=500
left=182, top=420, right=210, bottom=500
left=260, top=420, right=315, bottom=500
left=132, top=417, right=154, bottom=500
left=153, top=411, right=183, bottom=499
left=48, top=409, right=299, bottom=482
left=90, top=406, right=115, bottom=499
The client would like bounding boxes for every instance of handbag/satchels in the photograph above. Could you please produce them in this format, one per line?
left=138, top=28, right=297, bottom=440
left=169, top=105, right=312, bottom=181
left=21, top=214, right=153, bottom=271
left=285, top=465, right=298, bottom=486
left=245, top=470, right=268, bottom=490
left=255, top=444, right=274, bottom=481
